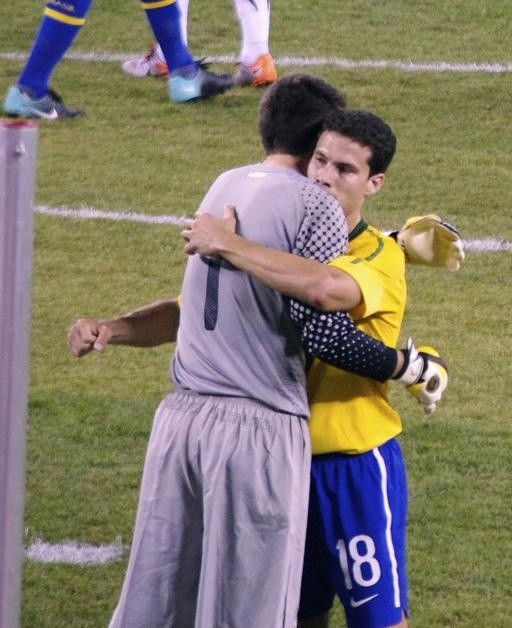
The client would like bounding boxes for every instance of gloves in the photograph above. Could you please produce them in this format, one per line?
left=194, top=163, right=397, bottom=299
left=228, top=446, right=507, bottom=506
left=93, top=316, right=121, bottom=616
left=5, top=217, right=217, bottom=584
left=388, top=215, right=465, bottom=272
left=392, top=344, right=448, bottom=415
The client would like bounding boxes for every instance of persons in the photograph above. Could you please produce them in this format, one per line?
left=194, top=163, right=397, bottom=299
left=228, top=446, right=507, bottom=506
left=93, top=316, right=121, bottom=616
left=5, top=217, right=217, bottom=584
left=64, top=105, right=414, bottom=627
left=0, top=0, right=250, bottom=125
left=120, top=0, right=277, bottom=87
left=107, top=73, right=464, bottom=627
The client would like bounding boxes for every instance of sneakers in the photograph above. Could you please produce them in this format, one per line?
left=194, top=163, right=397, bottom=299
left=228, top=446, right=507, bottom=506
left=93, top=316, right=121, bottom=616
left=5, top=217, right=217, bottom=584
left=235, top=53, right=278, bottom=86
left=122, top=45, right=234, bottom=102
left=4, top=86, right=84, bottom=120
